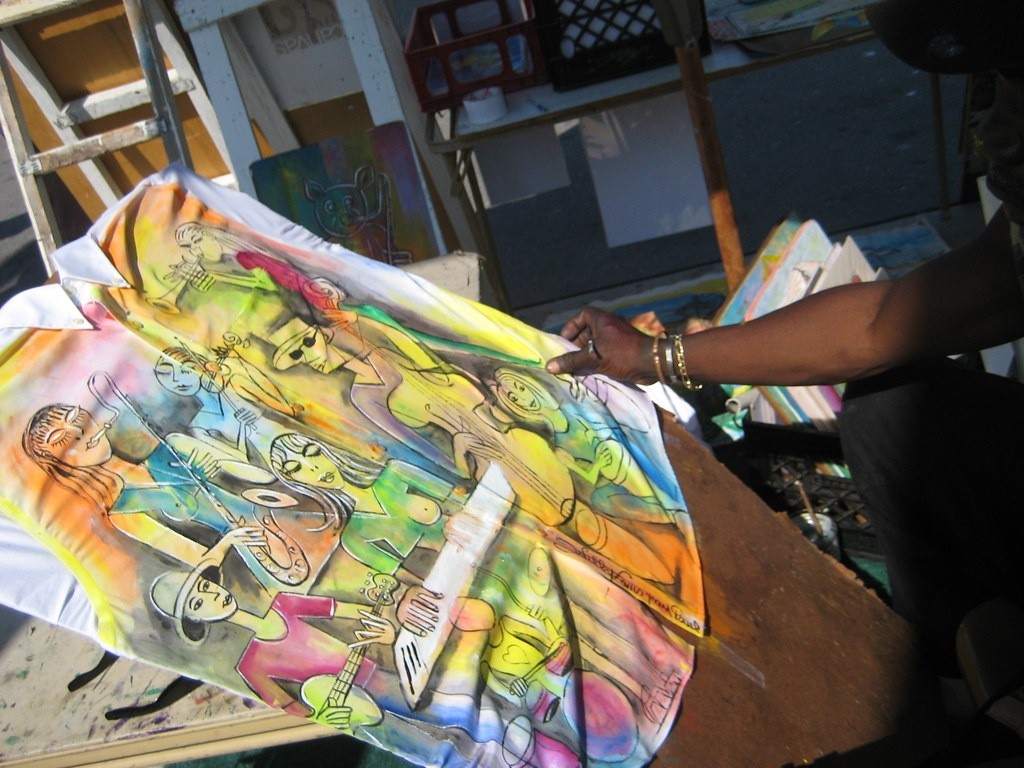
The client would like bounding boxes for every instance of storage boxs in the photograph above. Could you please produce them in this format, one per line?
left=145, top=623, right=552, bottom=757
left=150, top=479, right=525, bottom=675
left=695, top=385, right=882, bottom=557
left=532, top=0, right=711, bottom=92
left=403, top=0, right=548, bottom=114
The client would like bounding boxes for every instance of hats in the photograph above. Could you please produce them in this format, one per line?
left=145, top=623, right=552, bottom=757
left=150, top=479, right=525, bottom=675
left=864, top=1, right=1024, bottom=78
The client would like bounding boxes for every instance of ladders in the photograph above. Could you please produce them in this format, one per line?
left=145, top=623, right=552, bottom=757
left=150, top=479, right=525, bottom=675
left=0, top=0, right=240, bottom=279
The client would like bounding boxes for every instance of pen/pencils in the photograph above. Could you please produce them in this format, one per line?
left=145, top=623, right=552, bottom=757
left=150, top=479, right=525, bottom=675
left=522, top=94, right=548, bottom=113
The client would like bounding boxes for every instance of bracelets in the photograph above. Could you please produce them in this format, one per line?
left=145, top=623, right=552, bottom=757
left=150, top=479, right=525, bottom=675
left=653, top=331, right=703, bottom=392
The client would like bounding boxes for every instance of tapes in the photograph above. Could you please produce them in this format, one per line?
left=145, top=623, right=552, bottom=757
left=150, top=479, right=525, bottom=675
left=463, top=86, right=507, bottom=125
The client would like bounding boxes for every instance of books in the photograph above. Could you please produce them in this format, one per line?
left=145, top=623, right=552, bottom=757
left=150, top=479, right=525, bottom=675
left=741, top=219, right=890, bottom=479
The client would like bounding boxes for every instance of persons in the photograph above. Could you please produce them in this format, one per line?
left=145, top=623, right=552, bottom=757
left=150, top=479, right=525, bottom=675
left=546, top=0, right=1024, bottom=680
